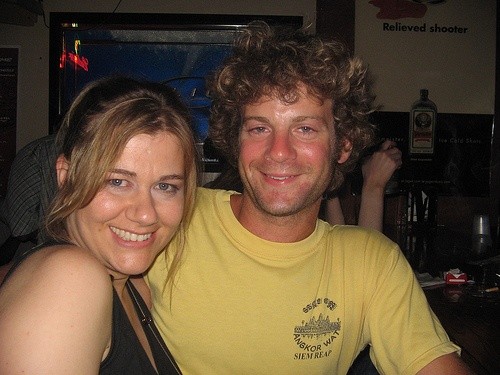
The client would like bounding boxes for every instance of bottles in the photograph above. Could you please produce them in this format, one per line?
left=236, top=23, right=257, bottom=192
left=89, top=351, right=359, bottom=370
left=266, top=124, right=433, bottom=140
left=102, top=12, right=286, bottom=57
left=409, top=88, right=438, bottom=154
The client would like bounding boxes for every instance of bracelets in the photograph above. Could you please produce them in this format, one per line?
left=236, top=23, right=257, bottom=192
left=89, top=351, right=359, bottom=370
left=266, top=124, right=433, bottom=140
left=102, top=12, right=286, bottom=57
left=326, top=190, right=339, bottom=200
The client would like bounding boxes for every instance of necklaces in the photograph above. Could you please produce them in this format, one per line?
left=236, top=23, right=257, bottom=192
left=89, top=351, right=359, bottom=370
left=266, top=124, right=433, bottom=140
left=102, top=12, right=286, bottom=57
left=126, top=280, right=181, bottom=375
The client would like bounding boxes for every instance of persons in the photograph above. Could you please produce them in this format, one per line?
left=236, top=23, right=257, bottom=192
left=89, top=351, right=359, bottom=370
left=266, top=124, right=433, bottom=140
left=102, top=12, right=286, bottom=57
left=0, top=116, right=402, bottom=269
left=144, top=20, right=475, bottom=375
left=0, top=76, right=203, bottom=375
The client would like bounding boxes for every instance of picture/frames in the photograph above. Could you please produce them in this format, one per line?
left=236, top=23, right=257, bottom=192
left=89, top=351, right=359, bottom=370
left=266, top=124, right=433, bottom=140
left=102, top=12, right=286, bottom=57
left=47, top=10, right=303, bottom=172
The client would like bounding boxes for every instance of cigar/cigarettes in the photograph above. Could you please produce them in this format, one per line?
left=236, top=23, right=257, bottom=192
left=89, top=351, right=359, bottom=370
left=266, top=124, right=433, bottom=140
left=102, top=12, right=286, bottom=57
left=486, top=287, right=500, bottom=292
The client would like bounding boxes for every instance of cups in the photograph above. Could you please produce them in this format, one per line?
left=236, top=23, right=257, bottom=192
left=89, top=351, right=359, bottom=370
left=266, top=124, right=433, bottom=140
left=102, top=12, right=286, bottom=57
left=471, top=212, right=493, bottom=246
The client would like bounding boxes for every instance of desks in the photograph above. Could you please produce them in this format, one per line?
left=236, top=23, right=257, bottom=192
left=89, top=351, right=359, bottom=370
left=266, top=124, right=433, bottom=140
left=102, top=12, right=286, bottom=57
left=348, top=215, right=499, bottom=375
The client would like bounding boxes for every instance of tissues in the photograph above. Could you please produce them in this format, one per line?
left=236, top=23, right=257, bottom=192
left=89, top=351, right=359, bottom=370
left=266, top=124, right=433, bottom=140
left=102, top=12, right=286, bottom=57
left=443, top=268, right=467, bottom=285
